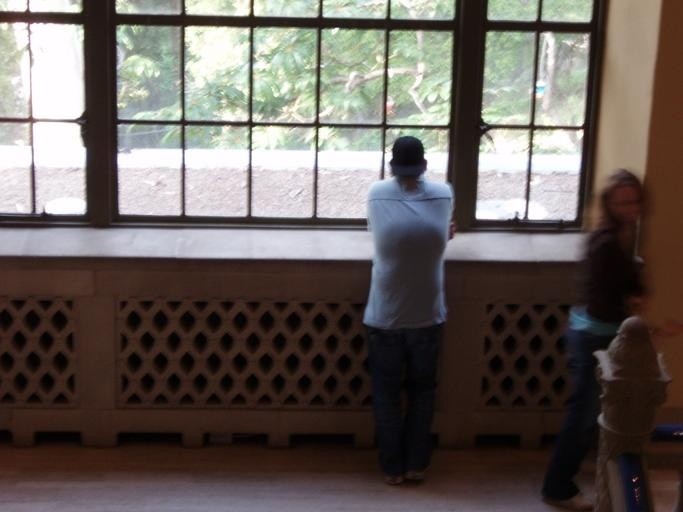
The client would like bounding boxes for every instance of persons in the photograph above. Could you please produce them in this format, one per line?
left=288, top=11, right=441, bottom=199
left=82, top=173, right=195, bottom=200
left=536, top=166, right=653, bottom=511
left=361, top=135, right=458, bottom=487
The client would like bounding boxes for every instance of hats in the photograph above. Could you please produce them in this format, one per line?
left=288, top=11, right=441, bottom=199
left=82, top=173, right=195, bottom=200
left=392, top=136, right=425, bottom=180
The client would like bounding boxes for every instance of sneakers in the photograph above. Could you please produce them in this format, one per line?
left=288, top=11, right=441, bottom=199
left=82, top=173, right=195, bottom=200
left=541, top=489, right=596, bottom=512
left=385, top=468, right=427, bottom=485
